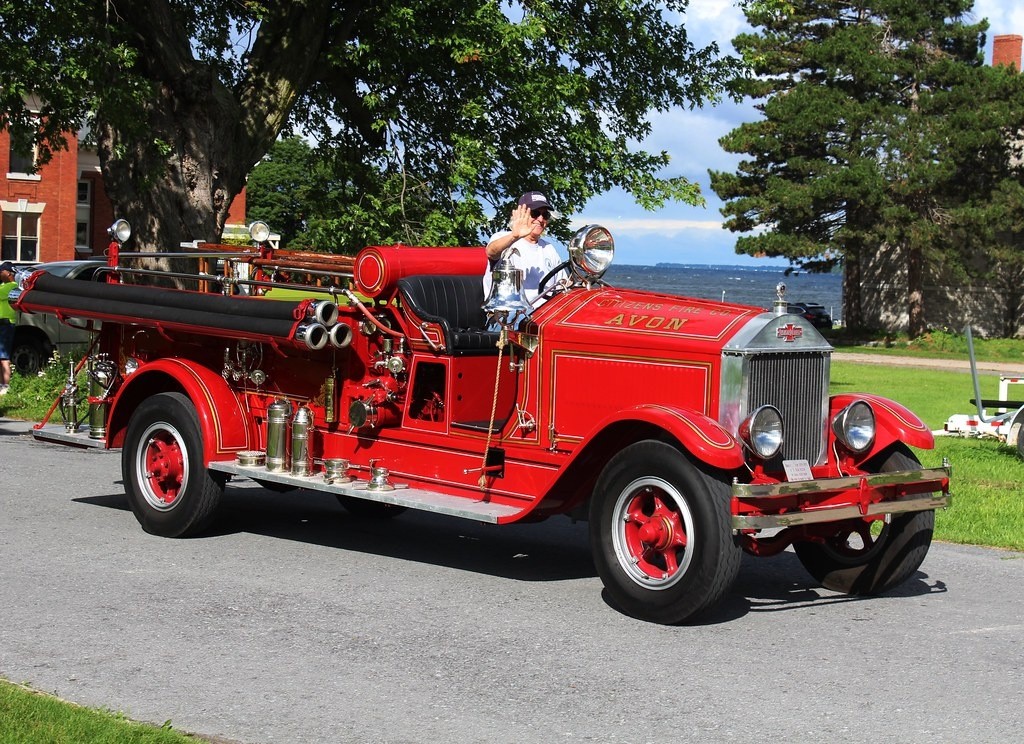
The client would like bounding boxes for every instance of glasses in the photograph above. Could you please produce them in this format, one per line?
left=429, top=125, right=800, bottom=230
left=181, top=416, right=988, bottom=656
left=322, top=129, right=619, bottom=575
left=528, top=210, right=551, bottom=220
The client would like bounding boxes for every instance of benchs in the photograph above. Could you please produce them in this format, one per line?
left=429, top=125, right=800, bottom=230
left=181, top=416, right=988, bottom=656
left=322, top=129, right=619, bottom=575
left=396, top=274, right=519, bottom=425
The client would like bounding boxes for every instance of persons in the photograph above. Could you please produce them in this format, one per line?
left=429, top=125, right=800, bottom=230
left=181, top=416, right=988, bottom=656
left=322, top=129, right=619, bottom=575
left=482, top=191, right=575, bottom=333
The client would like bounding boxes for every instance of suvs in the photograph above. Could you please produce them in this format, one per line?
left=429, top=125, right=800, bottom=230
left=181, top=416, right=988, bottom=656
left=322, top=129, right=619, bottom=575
left=786, top=301, right=833, bottom=330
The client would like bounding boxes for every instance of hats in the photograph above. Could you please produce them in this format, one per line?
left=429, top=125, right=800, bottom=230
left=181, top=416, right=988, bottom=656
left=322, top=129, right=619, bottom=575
left=519, top=191, right=555, bottom=212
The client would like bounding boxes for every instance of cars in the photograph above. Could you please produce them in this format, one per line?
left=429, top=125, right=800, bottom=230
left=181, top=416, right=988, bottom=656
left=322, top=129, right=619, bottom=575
left=8, top=260, right=108, bottom=377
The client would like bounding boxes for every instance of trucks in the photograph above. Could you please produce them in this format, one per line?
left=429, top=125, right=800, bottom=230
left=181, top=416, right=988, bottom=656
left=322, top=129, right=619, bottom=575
left=7, top=219, right=954, bottom=626
